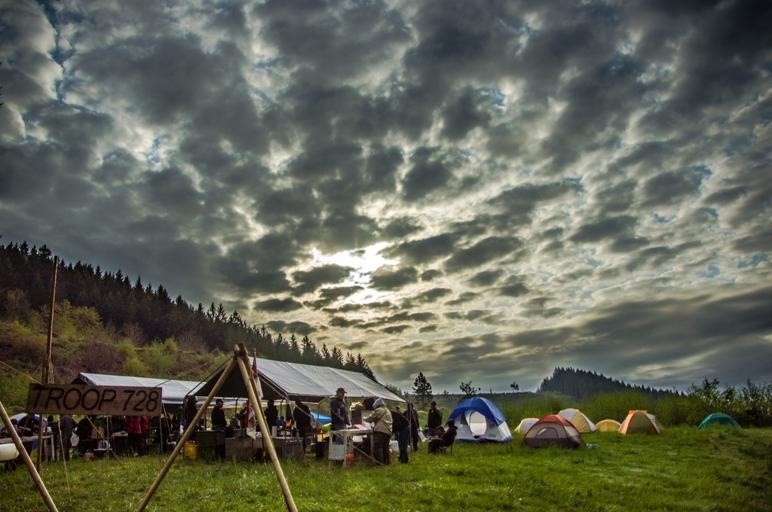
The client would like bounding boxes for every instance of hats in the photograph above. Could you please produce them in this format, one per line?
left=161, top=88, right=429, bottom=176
left=337, top=388, right=347, bottom=394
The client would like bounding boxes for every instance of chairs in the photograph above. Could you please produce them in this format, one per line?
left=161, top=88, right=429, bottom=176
left=435, top=433, right=456, bottom=456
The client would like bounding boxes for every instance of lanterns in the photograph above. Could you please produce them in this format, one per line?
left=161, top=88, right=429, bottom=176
left=556, top=407, right=597, bottom=433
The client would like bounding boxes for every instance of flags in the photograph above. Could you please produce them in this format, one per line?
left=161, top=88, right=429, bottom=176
left=245, top=354, right=263, bottom=441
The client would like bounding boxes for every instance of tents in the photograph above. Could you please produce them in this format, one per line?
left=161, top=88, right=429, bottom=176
left=442, top=396, right=514, bottom=442
left=593, top=418, right=622, bottom=432
left=617, top=409, right=660, bottom=435
left=511, top=416, right=540, bottom=435
left=522, top=414, right=588, bottom=449
left=696, top=411, right=741, bottom=430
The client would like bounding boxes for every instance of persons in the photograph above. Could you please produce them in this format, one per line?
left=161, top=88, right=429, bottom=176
left=329, top=387, right=350, bottom=440
left=427, top=400, right=442, bottom=427
left=211, top=399, right=228, bottom=464
left=427, top=419, right=458, bottom=453
left=390, top=410, right=411, bottom=465
left=362, top=397, right=394, bottom=467
left=2, top=398, right=208, bottom=472
left=228, top=398, right=315, bottom=455
left=402, top=402, right=420, bottom=451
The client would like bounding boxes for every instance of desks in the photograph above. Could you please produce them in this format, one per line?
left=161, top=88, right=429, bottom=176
left=328, top=429, right=375, bottom=468
left=194, top=429, right=304, bottom=462
left=0, top=435, right=55, bottom=462
